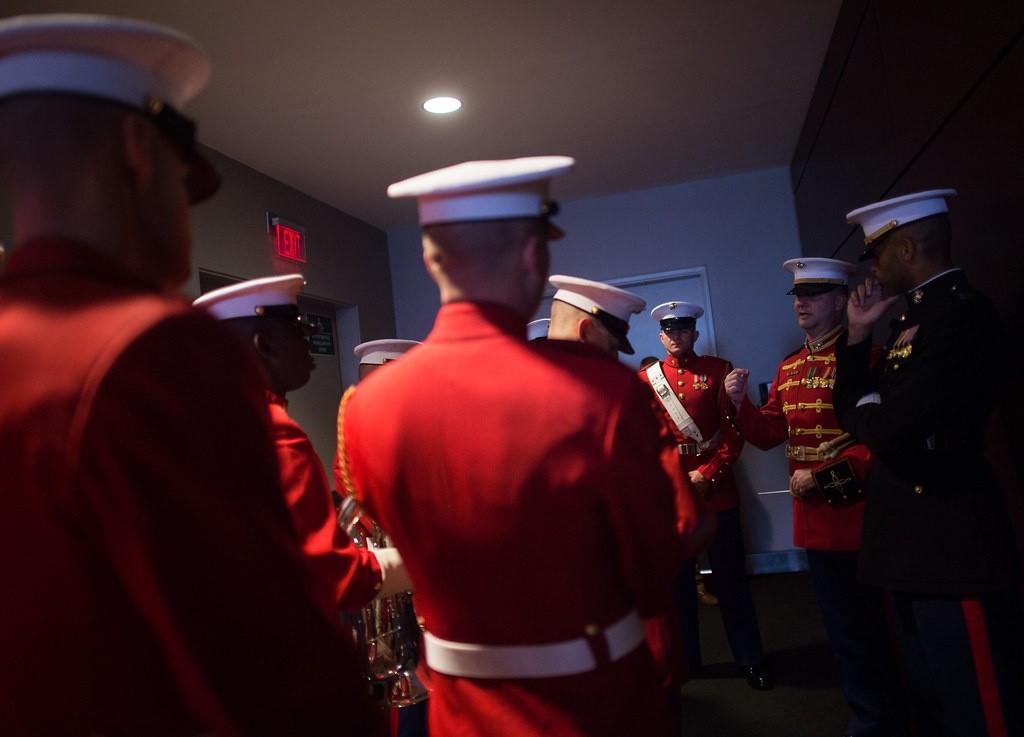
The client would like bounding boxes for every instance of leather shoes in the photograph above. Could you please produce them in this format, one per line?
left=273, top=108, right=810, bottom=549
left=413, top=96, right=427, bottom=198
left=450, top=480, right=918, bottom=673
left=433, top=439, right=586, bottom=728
left=736, top=655, right=773, bottom=690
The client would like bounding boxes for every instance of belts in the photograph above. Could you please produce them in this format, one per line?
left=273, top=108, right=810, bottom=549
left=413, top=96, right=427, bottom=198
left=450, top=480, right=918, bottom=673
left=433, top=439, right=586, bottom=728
left=679, top=442, right=713, bottom=455
left=421, top=608, right=641, bottom=679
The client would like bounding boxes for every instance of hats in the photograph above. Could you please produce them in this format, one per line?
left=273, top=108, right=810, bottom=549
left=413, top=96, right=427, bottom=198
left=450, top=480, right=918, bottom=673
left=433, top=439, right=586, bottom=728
left=191, top=273, right=321, bottom=335
left=0, top=13, right=224, bottom=207
left=650, top=301, right=704, bottom=330
left=548, top=275, right=646, bottom=355
left=354, top=339, right=421, bottom=365
left=783, top=257, right=851, bottom=296
left=844, top=188, right=957, bottom=260
left=387, top=155, right=576, bottom=240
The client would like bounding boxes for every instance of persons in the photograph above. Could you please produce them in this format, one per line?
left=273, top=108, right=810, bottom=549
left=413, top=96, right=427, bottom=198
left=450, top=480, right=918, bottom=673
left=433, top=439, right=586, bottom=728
left=192, top=274, right=424, bottom=632
left=336, top=156, right=762, bottom=737
left=723, top=256, right=883, bottom=667
left=832, top=187, right=1024, bottom=737
left=1, top=13, right=306, bottom=736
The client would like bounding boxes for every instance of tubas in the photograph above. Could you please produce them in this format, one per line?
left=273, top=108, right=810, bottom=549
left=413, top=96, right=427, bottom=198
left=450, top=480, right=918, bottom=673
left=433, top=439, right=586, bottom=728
left=338, top=495, right=429, bottom=710
